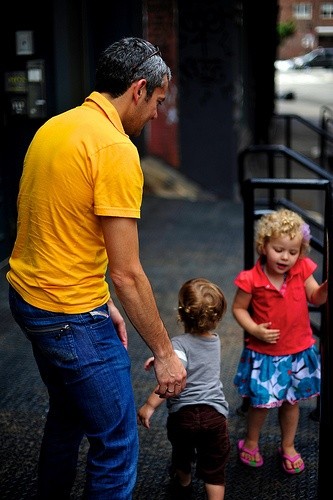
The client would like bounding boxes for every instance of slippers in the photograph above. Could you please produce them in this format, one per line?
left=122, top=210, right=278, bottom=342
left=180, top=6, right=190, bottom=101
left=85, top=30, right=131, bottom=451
left=278, top=448, right=304, bottom=473
left=237, top=439, right=263, bottom=467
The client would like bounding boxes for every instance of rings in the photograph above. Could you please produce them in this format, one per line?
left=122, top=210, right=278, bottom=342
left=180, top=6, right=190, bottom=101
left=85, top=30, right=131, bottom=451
left=167, top=390, right=174, bottom=393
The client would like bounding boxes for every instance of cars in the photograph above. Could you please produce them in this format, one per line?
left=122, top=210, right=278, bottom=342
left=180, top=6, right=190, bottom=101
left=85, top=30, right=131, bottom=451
left=274, top=48, right=333, bottom=103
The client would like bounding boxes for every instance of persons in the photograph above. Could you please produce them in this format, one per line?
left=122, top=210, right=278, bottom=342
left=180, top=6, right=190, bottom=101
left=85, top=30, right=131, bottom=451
left=232, top=208, right=328, bottom=473
left=137, top=277, right=229, bottom=500
left=5, top=37, right=187, bottom=500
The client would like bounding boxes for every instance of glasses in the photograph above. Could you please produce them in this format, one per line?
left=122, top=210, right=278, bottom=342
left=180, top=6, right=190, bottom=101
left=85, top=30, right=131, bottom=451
left=130, top=46, right=161, bottom=80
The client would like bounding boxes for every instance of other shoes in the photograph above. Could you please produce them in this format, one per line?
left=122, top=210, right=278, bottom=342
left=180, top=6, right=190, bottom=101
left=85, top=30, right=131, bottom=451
left=175, top=472, right=192, bottom=495
